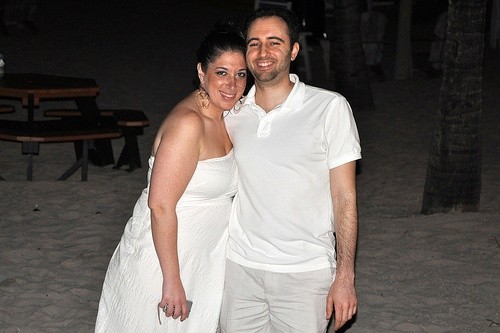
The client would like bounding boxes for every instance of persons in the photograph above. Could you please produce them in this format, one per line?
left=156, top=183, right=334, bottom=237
left=218, top=5, right=362, bottom=333
left=95, top=20, right=248, bottom=333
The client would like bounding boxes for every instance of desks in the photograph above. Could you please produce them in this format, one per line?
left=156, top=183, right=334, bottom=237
left=0, top=73, right=100, bottom=119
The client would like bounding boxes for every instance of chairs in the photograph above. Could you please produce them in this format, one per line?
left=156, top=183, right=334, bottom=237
left=254, top=0, right=313, bottom=81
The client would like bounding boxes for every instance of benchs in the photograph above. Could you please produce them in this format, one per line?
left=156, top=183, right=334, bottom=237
left=0, top=104, right=150, bottom=182
